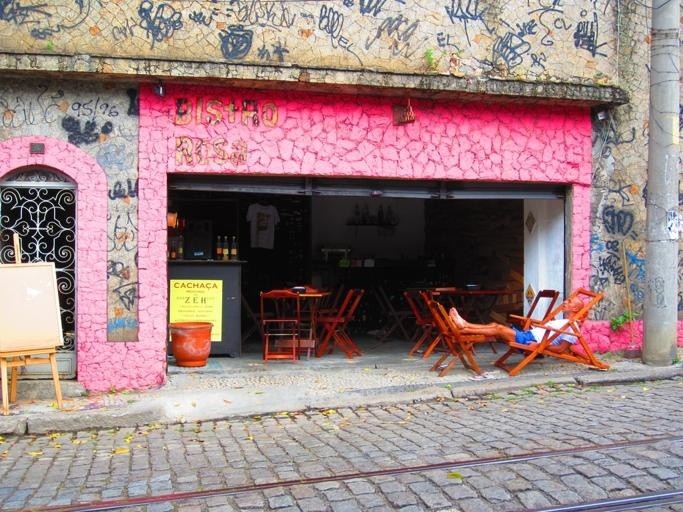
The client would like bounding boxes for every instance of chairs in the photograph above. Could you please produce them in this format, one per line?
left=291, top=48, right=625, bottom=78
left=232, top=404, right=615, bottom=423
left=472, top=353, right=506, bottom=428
left=403, top=288, right=611, bottom=378
left=241, top=284, right=412, bottom=364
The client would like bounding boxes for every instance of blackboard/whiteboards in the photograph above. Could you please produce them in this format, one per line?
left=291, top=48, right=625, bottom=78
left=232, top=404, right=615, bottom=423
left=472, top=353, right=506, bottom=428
left=0, top=261, right=64, bottom=353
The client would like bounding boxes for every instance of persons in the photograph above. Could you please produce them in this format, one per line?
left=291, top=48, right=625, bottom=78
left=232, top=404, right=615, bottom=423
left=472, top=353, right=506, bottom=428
left=448, top=296, right=584, bottom=357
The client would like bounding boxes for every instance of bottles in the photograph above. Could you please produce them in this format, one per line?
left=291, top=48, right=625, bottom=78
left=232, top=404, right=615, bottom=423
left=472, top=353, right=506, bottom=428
left=216, top=235, right=238, bottom=260
left=168, top=235, right=184, bottom=260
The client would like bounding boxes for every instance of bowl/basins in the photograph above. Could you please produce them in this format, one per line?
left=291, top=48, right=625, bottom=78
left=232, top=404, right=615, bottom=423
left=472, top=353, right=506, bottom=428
left=466, top=284, right=480, bottom=290
left=293, top=288, right=306, bottom=294
